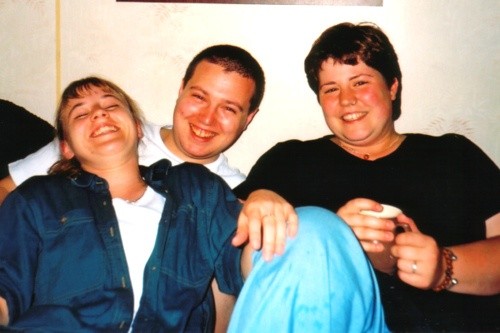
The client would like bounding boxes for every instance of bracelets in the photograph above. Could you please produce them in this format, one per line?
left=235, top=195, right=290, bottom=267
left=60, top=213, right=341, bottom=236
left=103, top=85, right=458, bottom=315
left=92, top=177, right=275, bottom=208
left=432, top=247, right=458, bottom=292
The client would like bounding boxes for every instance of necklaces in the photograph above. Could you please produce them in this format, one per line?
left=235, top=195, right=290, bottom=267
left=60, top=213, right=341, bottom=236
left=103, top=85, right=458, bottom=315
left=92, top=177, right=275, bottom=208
left=334, top=134, right=401, bottom=159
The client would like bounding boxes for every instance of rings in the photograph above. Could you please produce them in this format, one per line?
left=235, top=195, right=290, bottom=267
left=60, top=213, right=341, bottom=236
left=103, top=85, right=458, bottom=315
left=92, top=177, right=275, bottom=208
left=412, top=261, right=416, bottom=274
left=262, top=214, right=273, bottom=218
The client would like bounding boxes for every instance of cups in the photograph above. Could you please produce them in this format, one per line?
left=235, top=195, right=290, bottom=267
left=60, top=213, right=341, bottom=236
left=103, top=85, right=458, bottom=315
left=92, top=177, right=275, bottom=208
left=358, top=204, right=412, bottom=270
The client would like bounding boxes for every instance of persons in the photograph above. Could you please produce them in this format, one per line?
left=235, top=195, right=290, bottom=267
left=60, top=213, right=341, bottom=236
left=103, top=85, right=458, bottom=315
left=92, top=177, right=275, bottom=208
left=0, top=77, right=299, bottom=333
left=0, top=97, right=56, bottom=182
left=231, top=22, right=500, bottom=333
left=0, top=44, right=265, bottom=206
